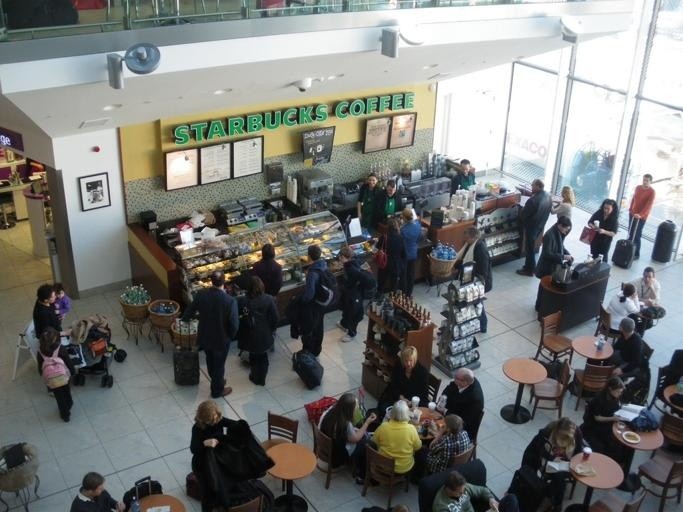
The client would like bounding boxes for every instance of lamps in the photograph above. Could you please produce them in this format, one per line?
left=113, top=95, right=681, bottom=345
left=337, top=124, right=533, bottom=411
left=107, top=43, right=161, bottom=89
left=382, top=17, right=425, bottom=58
left=560, top=15, right=592, bottom=43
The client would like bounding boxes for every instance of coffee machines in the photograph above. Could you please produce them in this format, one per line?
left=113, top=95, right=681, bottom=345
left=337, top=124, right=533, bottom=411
left=295, top=167, right=333, bottom=212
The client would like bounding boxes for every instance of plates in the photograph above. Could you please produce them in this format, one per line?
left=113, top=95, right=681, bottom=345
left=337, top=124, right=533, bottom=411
left=623, top=431, right=641, bottom=444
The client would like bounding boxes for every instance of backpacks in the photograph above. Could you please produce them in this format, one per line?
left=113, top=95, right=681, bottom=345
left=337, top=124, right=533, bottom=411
left=309, top=262, right=339, bottom=309
left=360, top=269, right=377, bottom=299
left=37, top=344, right=71, bottom=390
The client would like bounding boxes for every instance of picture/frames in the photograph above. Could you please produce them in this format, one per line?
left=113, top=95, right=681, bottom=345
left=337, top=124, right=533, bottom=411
left=301, top=125, right=336, bottom=167
left=362, top=112, right=417, bottom=154
left=78, top=172, right=111, bottom=211
left=163, top=135, right=263, bottom=191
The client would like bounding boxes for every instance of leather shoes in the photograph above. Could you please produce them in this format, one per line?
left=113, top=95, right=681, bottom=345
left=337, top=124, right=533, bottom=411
left=516, top=269, right=532, bottom=276
left=221, top=387, right=232, bottom=395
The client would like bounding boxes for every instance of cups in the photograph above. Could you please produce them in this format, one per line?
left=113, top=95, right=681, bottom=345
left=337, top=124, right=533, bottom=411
left=583, top=448, right=591, bottom=460
left=616, top=422, right=625, bottom=435
left=443, top=188, right=476, bottom=223
left=594, top=220, right=600, bottom=227
left=407, top=395, right=438, bottom=438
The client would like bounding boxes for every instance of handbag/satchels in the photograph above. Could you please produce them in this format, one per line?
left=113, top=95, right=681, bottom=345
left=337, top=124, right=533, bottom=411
left=579, top=226, right=597, bottom=245
left=376, top=247, right=388, bottom=270
left=645, top=306, right=665, bottom=319
left=630, top=409, right=659, bottom=431
left=239, top=312, right=257, bottom=333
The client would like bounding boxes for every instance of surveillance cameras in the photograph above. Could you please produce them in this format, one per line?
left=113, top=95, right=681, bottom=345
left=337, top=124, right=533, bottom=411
left=296, top=79, right=311, bottom=91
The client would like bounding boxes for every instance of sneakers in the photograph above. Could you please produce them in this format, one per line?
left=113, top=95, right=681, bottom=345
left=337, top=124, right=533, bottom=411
left=336, top=320, right=354, bottom=342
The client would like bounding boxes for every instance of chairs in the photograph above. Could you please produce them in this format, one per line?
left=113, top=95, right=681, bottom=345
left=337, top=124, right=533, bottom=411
left=209, top=410, right=316, bottom=512
left=500, top=298, right=683, bottom=511
left=0, top=440, right=43, bottom=512
left=314, top=372, right=494, bottom=511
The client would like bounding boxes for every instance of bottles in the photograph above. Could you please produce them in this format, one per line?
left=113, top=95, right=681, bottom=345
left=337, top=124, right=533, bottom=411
left=678, top=377, right=683, bottom=394
left=368, top=158, right=389, bottom=184
left=596, top=337, right=605, bottom=352
left=584, top=254, right=593, bottom=264
left=131, top=497, right=140, bottom=512
left=598, top=254, right=603, bottom=266
left=431, top=240, right=455, bottom=261
left=363, top=290, right=431, bottom=383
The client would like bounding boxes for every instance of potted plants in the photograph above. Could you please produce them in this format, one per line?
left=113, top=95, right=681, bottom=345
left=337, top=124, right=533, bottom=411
left=119, top=284, right=180, bottom=326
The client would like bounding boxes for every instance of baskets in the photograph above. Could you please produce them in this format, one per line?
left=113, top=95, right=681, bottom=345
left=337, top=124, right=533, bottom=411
left=118, top=294, right=198, bottom=346
left=427, top=252, right=457, bottom=278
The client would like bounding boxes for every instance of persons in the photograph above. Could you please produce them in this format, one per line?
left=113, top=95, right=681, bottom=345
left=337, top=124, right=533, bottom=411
left=70, top=472, right=126, bottom=512
left=181, top=158, right=683, bottom=511
left=53, top=283, right=72, bottom=318
left=33, top=283, right=73, bottom=376
left=36, top=325, right=76, bottom=423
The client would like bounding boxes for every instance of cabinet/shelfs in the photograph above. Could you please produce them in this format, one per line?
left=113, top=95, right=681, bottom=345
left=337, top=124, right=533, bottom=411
left=174, top=210, right=349, bottom=304
left=0, top=157, right=31, bottom=221
left=22, top=184, right=55, bottom=258
left=433, top=262, right=487, bottom=379
left=475, top=202, right=525, bottom=267
left=362, top=292, right=435, bottom=402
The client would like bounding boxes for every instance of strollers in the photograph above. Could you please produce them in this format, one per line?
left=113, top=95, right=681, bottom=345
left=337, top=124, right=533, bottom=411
left=57, top=322, right=127, bottom=388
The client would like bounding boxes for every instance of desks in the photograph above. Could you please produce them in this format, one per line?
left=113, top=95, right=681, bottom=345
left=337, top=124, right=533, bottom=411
left=535, top=262, right=611, bottom=333
left=425, top=275, right=454, bottom=298
left=126, top=493, right=187, bottom=512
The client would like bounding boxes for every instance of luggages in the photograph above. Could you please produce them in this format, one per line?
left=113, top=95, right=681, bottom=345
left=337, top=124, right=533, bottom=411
left=174, top=319, right=199, bottom=385
left=611, top=215, right=642, bottom=269
left=272, top=332, right=323, bottom=389
left=507, top=437, right=552, bottom=512
left=123, top=476, right=163, bottom=510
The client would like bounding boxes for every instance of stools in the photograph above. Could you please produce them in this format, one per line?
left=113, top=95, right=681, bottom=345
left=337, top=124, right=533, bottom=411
left=0, top=202, right=17, bottom=230
